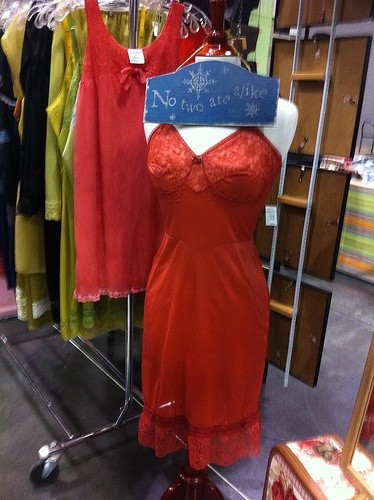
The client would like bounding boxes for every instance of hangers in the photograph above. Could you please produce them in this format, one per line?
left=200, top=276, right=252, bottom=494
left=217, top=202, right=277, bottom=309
left=0, top=0, right=212, bottom=39
